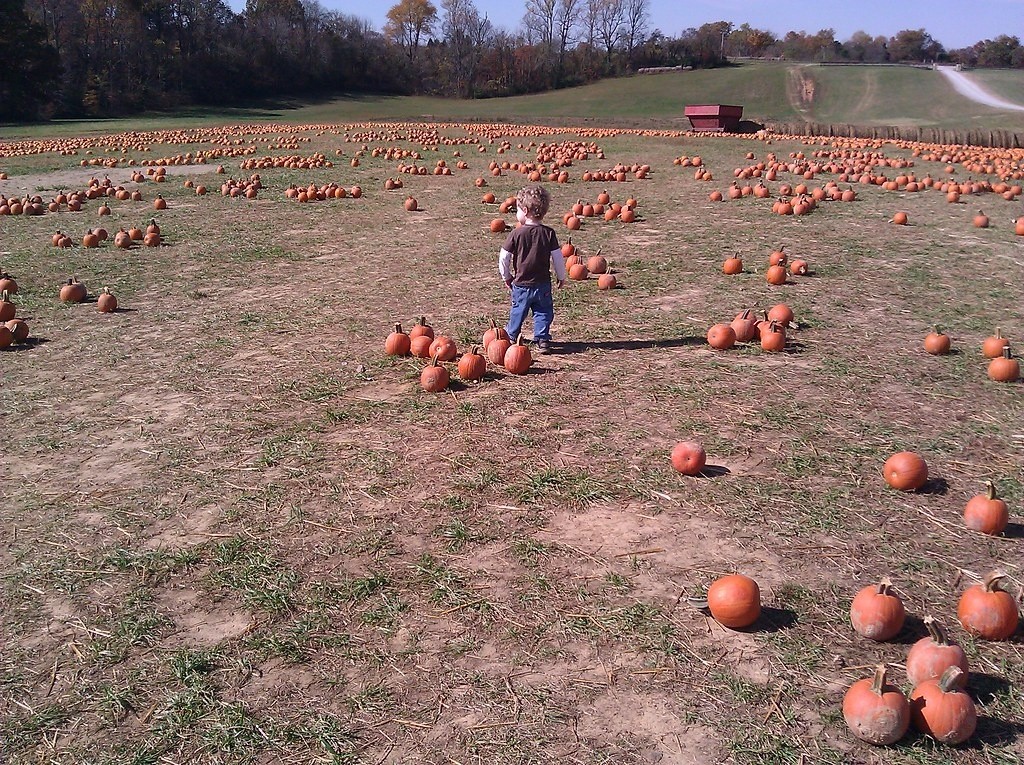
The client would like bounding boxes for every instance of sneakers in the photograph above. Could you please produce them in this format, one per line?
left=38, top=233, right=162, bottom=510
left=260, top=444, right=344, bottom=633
left=502, top=326, right=516, bottom=344
left=534, top=338, right=550, bottom=354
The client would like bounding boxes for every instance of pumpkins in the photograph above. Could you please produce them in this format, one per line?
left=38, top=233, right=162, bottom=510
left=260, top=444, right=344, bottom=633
left=850, top=575, right=906, bottom=641
left=672, top=443, right=706, bottom=475
left=957, top=570, right=1019, bottom=640
left=907, top=615, right=970, bottom=690
left=884, top=451, right=928, bottom=490
left=842, top=665, right=910, bottom=745
left=686, top=575, right=761, bottom=628
left=0, top=123, right=1024, bottom=392
left=963, top=481, right=1009, bottom=534
left=910, top=665, right=977, bottom=747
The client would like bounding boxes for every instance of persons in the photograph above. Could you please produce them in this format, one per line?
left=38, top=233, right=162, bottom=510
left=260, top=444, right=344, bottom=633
left=498, top=185, right=566, bottom=349
